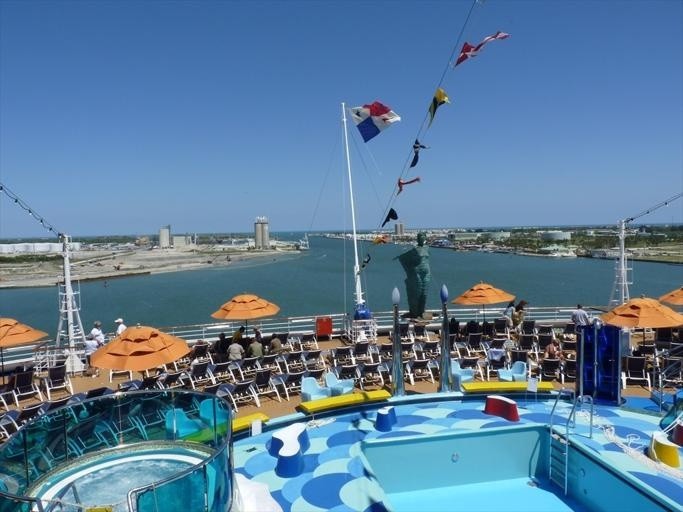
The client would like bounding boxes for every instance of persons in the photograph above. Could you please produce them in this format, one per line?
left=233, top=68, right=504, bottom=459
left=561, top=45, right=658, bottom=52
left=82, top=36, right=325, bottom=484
left=82, top=335, right=98, bottom=377
left=113, top=317, right=126, bottom=337
left=572, top=302, right=591, bottom=326
left=190, top=324, right=280, bottom=360
left=89, top=320, right=103, bottom=346
left=504, top=299, right=521, bottom=320
left=515, top=299, right=527, bottom=320
left=645, top=354, right=662, bottom=374
left=393, top=230, right=430, bottom=315
left=543, top=339, right=559, bottom=358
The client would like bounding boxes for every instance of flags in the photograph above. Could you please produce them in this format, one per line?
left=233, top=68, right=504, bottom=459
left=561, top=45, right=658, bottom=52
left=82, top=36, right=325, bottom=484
left=423, top=88, right=452, bottom=128
left=409, top=139, right=430, bottom=168
left=454, top=31, right=512, bottom=68
left=347, top=100, right=401, bottom=143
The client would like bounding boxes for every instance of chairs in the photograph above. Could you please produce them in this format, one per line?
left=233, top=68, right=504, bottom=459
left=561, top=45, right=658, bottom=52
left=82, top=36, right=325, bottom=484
left=0, top=364, right=191, bottom=486
left=193, top=319, right=577, bottom=412
left=621, top=335, right=682, bottom=393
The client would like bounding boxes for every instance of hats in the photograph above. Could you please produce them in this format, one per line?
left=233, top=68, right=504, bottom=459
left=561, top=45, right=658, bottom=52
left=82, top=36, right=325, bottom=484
left=114, top=318, right=123, bottom=323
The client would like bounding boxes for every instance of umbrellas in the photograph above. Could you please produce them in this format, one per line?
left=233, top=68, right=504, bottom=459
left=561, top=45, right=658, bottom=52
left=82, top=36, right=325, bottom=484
left=394, top=177, right=421, bottom=196
left=361, top=254, right=371, bottom=269
left=0, top=317, right=47, bottom=387
left=210, top=291, right=279, bottom=336
left=373, top=235, right=387, bottom=245
left=90, top=322, right=192, bottom=375
left=451, top=280, right=516, bottom=324
left=598, top=294, right=683, bottom=355
left=658, top=286, right=683, bottom=306
left=381, top=208, right=398, bottom=228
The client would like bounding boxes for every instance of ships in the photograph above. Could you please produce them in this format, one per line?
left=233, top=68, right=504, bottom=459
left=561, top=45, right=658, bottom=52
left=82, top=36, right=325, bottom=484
left=0, top=280, right=682, bottom=510
